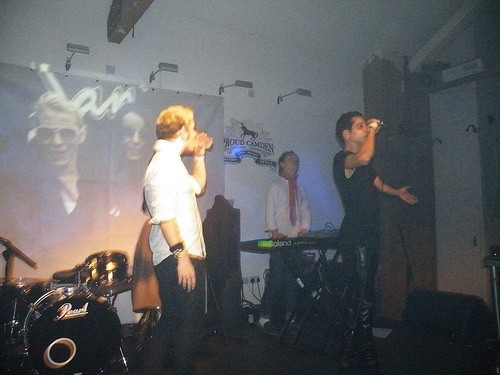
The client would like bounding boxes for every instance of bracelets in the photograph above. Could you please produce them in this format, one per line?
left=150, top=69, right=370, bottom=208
left=169, top=242, right=184, bottom=257
left=367, top=122, right=380, bottom=130
left=194, top=154, right=205, bottom=161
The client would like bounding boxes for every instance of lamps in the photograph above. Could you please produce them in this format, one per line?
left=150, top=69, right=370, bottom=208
left=149, top=63, right=178, bottom=83
left=65, top=43, right=89, bottom=70
left=277, top=88, right=312, bottom=104
left=219, top=80, right=254, bottom=95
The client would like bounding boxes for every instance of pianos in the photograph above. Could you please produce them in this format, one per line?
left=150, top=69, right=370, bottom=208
left=240, top=237, right=341, bottom=344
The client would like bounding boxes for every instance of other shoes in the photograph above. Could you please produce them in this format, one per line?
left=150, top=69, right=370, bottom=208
left=361, top=365, right=386, bottom=375
left=285, top=320, right=301, bottom=335
left=254, top=319, right=278, bottom=335
left=337, top=368, right=356, bottom=375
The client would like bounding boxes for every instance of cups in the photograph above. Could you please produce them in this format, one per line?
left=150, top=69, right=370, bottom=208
left=196, top=133, right=214, bottom=150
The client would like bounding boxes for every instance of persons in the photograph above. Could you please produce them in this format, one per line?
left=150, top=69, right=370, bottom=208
left=142, top=104, right=214, bottom=375
left=332, top=111, right=418, bottom=375
left=12, top=90, right=157, bottom=260
left=254, top=151, right=311, bottom=336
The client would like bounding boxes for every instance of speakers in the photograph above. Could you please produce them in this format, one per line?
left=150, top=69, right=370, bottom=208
left=401, top=286, right=497, bottom=344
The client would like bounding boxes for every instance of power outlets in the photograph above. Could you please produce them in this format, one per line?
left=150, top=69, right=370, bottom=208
left=250, top=276, right=260, bottom=283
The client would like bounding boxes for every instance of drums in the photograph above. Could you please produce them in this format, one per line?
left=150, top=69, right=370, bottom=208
left=83, top=250, right=130, bottom=295
left=0, top=277, right=123, bottom=375
